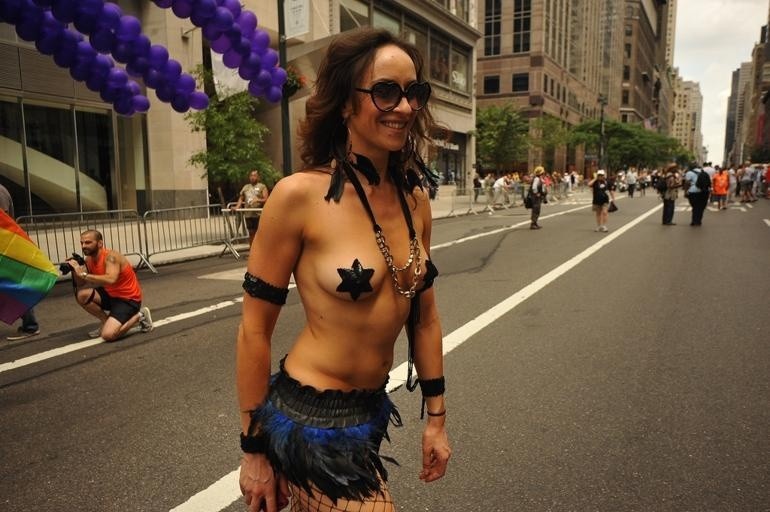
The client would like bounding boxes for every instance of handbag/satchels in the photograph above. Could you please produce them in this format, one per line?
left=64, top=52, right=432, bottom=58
left=524, top=195, right=531, bottom=210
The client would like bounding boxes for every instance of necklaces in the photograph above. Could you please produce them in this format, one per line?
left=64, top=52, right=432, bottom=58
left=321, top=148, right=422, bottom=299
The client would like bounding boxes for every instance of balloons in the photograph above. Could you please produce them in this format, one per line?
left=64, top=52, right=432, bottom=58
left=0, top=0, right=285, bottom=116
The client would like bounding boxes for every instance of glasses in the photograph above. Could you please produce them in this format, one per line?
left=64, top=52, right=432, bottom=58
left=353, top=79, right=433, bottom=112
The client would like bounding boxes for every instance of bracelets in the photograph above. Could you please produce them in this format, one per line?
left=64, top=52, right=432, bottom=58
left=427, top=411, right=446, bottom=416
left=239, top=432, right=267, bottom=455
left=419, top=376, right=446, bottom=397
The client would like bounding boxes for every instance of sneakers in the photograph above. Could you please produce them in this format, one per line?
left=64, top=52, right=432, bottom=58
left=138, top=306, right=153, bottom=334
left=5, top=324, right=40, bottom=341
left=600, top=226, right=610, bottom=233
left=87, top=327, right=102, bottom=339
left=594, top=224, right=600, bottom=233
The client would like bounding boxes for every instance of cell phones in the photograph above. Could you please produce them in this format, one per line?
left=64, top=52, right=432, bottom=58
left=260, top=487, right=289, bottom=512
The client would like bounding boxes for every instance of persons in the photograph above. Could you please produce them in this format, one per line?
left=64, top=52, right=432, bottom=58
left=471, top=157, right=770, bottom=234
left=56, top=231, right=154, bottom=343
left=0, top=184, right=41, bottom=340
left=240, top=26, right=459, bottom=511
left=232, top=170, right=268, bottom=249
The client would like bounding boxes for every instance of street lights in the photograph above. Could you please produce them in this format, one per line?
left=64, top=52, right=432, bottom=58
left=596, top=90, right=610, bottom=170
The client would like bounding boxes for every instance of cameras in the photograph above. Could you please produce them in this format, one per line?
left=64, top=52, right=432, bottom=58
left=60, top=252, right=85, bottom=275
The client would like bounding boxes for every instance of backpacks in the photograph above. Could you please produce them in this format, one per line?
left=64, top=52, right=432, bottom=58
left=690, top=168, right=711, bottom=190
left=657, top=173, right=673, bottom=194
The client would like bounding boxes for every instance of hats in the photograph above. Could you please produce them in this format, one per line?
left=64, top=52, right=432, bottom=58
left=596, top=169, right=606, bottom=177
left=533, top=165, right=545, bottom=175
left=667, top=161, right=677, bottom=171
